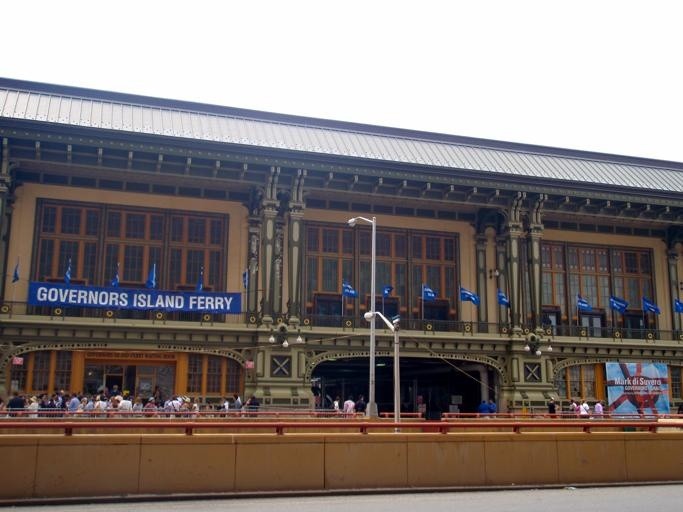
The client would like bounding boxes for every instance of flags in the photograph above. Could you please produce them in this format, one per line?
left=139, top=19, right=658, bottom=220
left=382, top=286, right=394, bottom=298
left=11, top=256, right=248, bottom=292
left=460, top=286, right=480, bottom=305
left=577, top=293, right=593, bottom=311
left=675, top=298, right=683, bottom=313
left=497, top=288, right=511, bottom=308
left=342, top=279, right=358, bottom=298
left=609, top=296, right=629, bottom=314
left=642, top=297, right=661, bottom=314
left=423, top=285, right=438, bottom=305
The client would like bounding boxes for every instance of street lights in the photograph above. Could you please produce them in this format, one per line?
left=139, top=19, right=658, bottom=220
left=362, top=309, right=404, bottom=432
left=346, top=214, right=382, bottom=420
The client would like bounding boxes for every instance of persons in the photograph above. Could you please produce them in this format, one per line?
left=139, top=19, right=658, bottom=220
left=547, top=397, right=560, bottom=413
left=343, top=395, right=356, bottom=412
left=488, top=399, right=497, bottom=418
left=355, top=395, right=366, bottom=412
left=570, top=398, right=604, bottom=419
left=478, top=400, right=489, bottom=418
left=333, top=396, right=342, bottom=409
left=0, top=384, right=261, bottom=419
